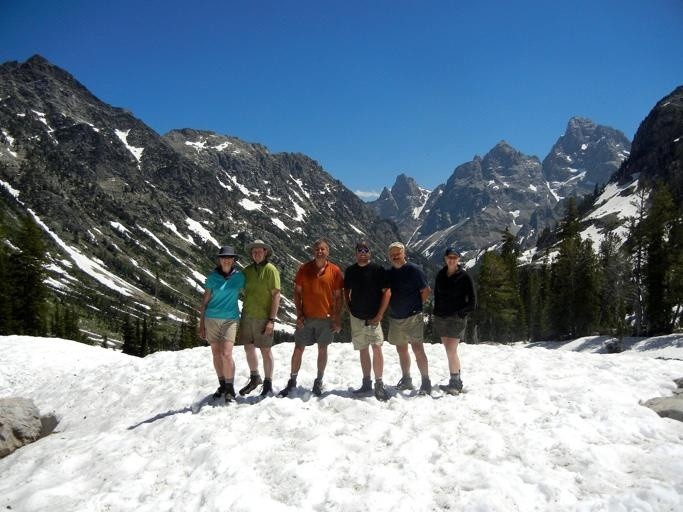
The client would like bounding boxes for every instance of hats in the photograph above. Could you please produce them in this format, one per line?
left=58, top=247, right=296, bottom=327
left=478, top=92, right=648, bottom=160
left=216, top=246, right=238, bottom=257
left=444, top=247, right=459, bottom=256
left=244, top=239, right=272, bottom=258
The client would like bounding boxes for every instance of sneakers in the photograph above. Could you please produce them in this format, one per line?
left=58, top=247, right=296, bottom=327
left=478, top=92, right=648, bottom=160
left=239, top=375, right=262, bottom=395
left=374, top=382, right=388, bottom=401
left=352, top=380, right=372, bottom=393
left=394, top=375, right=462, bottom=395
left=278, top=380, right=296, bottom=397
left=212, top=380, right=225, bottom=402
left=225, top=383, right=236, bottom=401
left=313, top=379, right=321, bottom=395
left=261, top=380, right=272, bottom=395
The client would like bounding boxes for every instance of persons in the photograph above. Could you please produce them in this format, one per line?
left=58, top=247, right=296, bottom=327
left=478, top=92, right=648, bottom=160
left=343, top=241, right=392, bottom=398
left=240, top=240, right=281, bottom=397
left=199, top=246, right=247, bottom=403
left=385, top=242, right=432, bottom=394
left=278, top=240, right=343, bottom=397
left=433, top=247, right=477, bottom=395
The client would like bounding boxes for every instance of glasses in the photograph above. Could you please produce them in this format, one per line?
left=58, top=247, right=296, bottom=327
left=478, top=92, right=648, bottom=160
left=358, top=248, right=369, bottom=252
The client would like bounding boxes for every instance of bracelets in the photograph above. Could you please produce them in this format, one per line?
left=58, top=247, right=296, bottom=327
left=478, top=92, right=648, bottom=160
left=269, top=318, right=275, bottom=323
